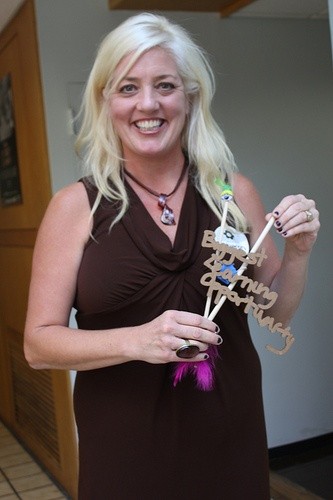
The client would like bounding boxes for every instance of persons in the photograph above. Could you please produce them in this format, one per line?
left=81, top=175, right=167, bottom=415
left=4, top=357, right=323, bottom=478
left=24, top=11, right=319, bottom=500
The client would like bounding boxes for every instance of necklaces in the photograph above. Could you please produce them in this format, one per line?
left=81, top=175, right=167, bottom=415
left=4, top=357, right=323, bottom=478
left=122, top=164, right=190, bottom=227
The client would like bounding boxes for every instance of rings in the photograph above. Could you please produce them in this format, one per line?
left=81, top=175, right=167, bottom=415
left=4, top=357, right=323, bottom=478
left=305, top=209, right=313, bottom=221
left=176, top=339, right=199, bottom=359
